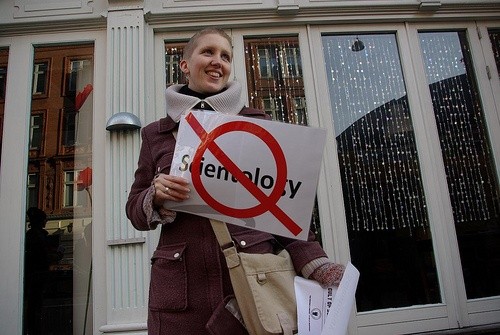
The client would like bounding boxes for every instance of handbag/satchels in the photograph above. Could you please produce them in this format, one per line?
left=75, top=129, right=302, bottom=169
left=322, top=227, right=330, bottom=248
left=223, top=246, right=299, bottom=335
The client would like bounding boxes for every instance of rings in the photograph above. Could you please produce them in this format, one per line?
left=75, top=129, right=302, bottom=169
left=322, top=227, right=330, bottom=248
left=164, top=187, right=169, bottom=194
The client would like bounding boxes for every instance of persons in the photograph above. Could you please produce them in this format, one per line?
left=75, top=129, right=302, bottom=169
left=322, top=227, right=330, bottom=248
left=125, top=28, right=345, bottom=335
left=22, top=206, right=65, bottom=335
